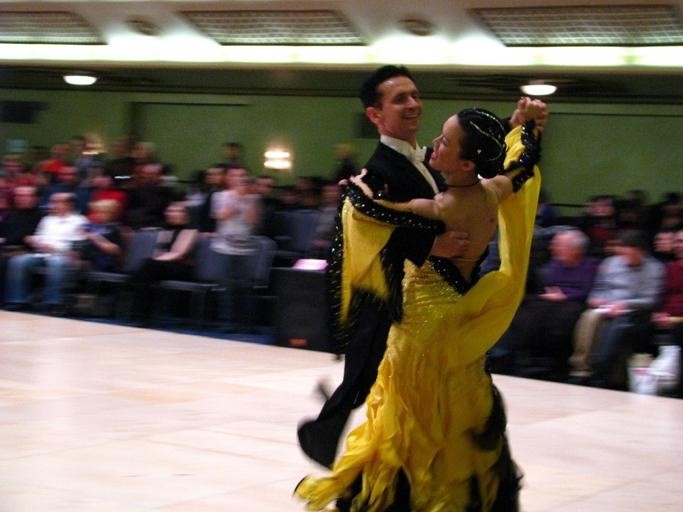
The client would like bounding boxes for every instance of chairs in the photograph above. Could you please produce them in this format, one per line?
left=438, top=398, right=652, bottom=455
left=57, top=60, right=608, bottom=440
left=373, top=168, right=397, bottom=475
left=1, top=146, right=339, bottom=336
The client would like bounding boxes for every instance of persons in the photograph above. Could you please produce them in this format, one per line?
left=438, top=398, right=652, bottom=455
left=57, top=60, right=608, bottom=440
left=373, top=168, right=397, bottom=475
left=295, top=64, right=547, bottom=511
left=0, top=133, right=683, bottom=395
